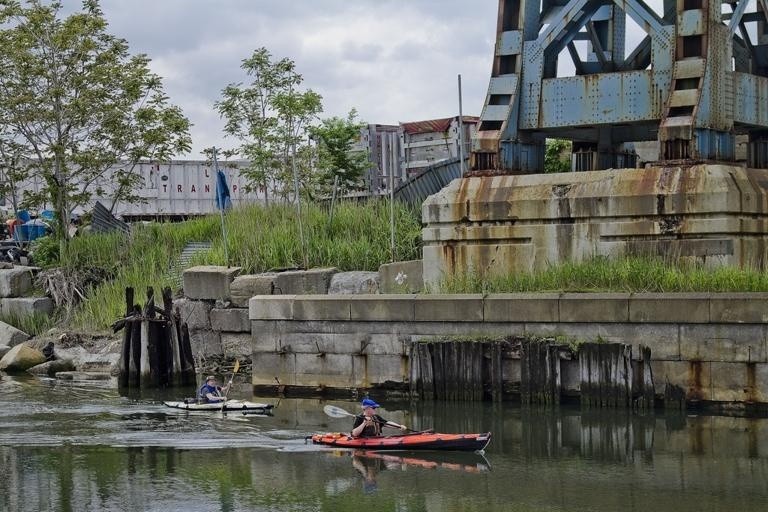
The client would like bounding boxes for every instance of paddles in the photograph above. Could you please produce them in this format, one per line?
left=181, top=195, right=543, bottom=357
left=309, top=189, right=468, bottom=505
left=323, top=404, right=418, bottom=433
left=220, top=359, right=239, bottom=411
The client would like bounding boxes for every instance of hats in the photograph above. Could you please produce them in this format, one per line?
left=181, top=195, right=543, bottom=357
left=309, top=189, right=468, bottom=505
left=362, top=398, right=380, bottom=407
left=206, top=376, right=217, bottom=383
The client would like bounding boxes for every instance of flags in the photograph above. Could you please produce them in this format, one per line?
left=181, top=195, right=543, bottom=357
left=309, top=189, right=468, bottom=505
left=213, top=158, right=234, bottom=212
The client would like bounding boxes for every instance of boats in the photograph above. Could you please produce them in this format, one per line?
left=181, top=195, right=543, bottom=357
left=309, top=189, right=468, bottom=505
left=352, top=446, right=494, bottom=480
left=162, top=398, right=273, bottom=412
left=306, top=427, right=493, bottom=455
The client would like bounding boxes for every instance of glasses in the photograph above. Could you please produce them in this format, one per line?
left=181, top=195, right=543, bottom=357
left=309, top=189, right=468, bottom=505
left=365, top=407, right=376, bottom=410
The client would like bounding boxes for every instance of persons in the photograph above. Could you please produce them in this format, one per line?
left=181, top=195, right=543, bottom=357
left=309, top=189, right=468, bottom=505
left=195, top=375, right=233, bottom=404
left=347, top=399, right=407, bottom=437
left=23, top=211, right=43, bottom=225
left=347, top=453, right=405, bottom=495
